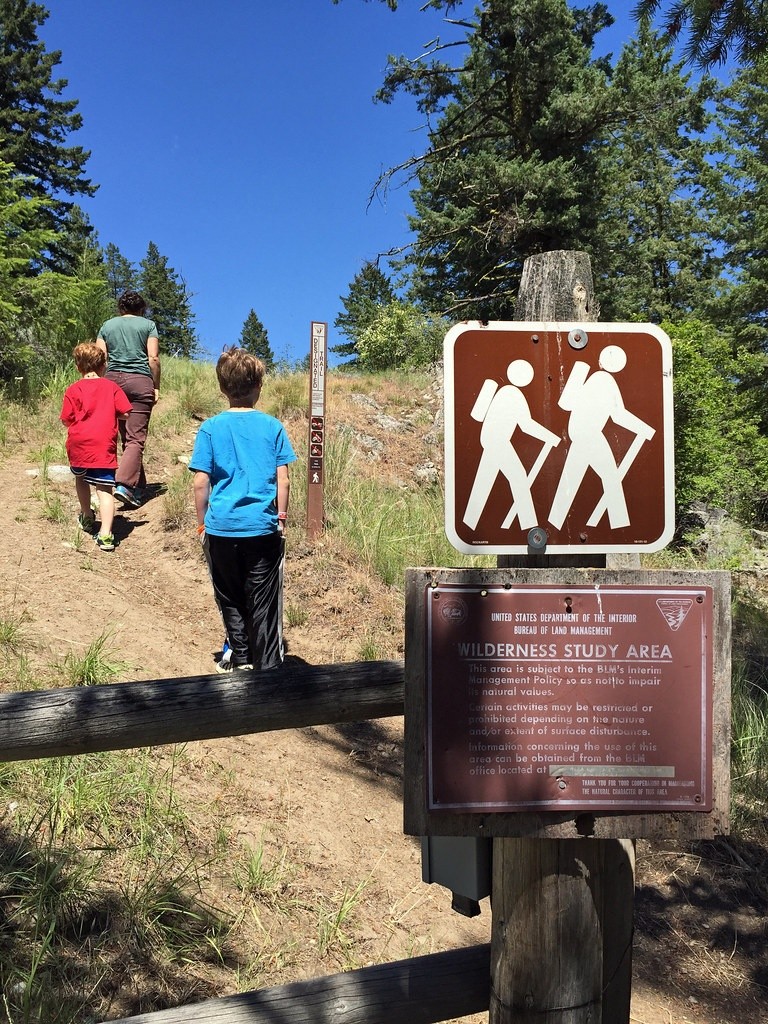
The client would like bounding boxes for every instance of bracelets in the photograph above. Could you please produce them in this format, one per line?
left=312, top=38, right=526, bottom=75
left=198, top=524, right=205, bottom=533
left=278, top=512, right=288, bottom=519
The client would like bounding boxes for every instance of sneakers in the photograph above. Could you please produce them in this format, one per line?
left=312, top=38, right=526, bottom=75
left=134, top=487, right=144, bottom=499
left=92, top=532, right=115, bottom=550
left=78, top=509, right=96, bottom=531
left=216, top=659, right=255, bottom=675
left=113, top=485, right=141, bottom=508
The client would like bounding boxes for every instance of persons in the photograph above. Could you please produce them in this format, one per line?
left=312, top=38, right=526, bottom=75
left=188, top=346, right=298, bottom=677
left=60, top=342, right=133, bottom=551
left=94, top=289, right=162, bottom=508
